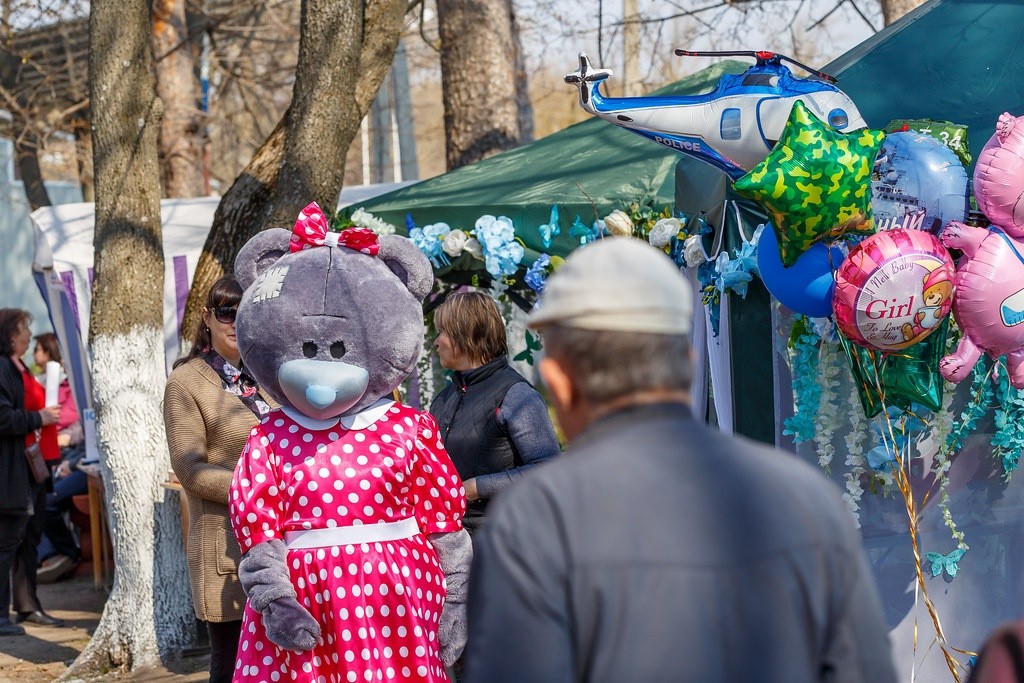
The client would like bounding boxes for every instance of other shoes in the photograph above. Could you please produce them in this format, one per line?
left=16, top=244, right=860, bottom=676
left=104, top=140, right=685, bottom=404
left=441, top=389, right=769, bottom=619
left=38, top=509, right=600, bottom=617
left=36, top=554, right=80, bottom=583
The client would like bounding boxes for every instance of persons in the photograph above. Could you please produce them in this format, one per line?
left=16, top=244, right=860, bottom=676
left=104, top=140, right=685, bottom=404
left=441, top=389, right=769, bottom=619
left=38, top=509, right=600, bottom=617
left=424, top=289, right=561, bottom=683
left=0, top=308, right=90, bottom=636
left=164, top=276, right=283, bottom=683
left=462, top=234, right=903, bottom=683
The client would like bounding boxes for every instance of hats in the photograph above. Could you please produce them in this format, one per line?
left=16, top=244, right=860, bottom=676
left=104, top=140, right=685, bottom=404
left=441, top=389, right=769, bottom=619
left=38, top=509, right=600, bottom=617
left=525, top=235, right=693, bottom=335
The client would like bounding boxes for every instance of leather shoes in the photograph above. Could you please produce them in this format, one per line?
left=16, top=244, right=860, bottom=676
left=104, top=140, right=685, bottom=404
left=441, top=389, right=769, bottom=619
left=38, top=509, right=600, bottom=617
left=0, top=618, right=25, bottom=635
left=16, top=610, right=64, bottom=627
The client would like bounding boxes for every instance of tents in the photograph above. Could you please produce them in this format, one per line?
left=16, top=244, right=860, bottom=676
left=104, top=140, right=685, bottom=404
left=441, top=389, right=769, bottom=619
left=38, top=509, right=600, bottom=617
left=31, top=0, right=805, bottom=452
left=806, top=0, right=1024, bottom=449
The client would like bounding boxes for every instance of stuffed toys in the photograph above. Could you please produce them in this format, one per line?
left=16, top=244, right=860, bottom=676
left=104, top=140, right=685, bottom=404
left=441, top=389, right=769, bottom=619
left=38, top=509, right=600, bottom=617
left=229, top=201, right=474, bottom=683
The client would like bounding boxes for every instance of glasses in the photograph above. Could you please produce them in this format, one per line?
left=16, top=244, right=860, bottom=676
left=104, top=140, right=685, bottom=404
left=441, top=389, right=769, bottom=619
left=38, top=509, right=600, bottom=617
left=208, top=306, right=237, bottom=324
left=34, top=348, right=40, bottom=353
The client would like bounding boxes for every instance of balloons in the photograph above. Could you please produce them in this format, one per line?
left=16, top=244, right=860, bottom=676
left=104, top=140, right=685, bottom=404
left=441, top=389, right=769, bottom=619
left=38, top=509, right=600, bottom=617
left=562, top=46, right=1024, bottom=419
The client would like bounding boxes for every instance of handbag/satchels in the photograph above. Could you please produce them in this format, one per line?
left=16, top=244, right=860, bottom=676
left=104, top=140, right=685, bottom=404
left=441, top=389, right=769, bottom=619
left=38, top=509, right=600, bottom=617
left=25, top=443, right=50, bottom=484
left=57, top=431, right=71, bottom=447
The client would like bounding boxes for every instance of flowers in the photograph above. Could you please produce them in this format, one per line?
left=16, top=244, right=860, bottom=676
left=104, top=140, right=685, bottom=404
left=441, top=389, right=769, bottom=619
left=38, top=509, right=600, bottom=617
left=352, top=207, right=1024, bottom=579
